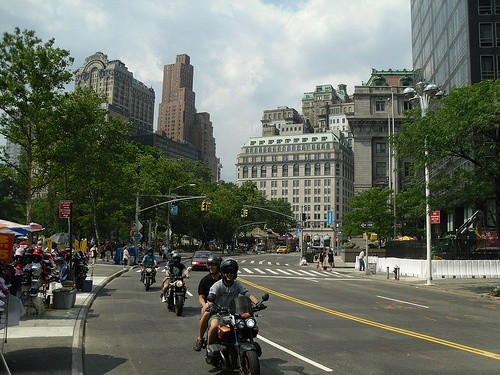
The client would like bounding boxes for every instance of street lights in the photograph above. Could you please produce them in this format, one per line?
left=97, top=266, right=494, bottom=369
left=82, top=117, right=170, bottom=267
left=402, top=81, right=444, bottom=286
left=167, top=183, right=197, bottom=252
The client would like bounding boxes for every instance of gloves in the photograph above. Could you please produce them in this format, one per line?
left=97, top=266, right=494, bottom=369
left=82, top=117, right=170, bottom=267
left=209, top=304, right=217, bottom=313
left=256, top=302, right=265, bottom=310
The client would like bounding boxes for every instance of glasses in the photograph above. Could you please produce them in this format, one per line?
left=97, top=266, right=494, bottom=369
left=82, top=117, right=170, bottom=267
left=207, top=263, right=216, bottom=267
left=222, top=270, right=234, bottom=274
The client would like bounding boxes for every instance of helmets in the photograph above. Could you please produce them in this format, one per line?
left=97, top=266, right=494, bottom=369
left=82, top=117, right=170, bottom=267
left=172, top=254, right=181, bottom=259
left=147, top=247, right=153, bottom=255
left=207, top=253, right=223, bottom=264
left=170, top=250, right=179, bottom=255
left=220, top=258, right=238, bottom=272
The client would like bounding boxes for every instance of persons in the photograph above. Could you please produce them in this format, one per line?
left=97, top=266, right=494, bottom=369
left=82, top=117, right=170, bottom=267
left=317, top=247, right=334, bottom=272
left=202, top=259, right=267, bottom=364
left=140, top=246, right=157, bottom=283
left=160, top=250, right=191, bottom=302
left=193, top=254, right=224, bottom=351
left=88, top=242, right=114, bottom=263
left=358, top=248, right=365, bottom=271
left=123, top=246, right=131, bottom=268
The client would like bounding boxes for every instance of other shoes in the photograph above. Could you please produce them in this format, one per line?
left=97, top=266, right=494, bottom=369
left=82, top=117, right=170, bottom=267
left=205, top=344, right=218, bottom=356
left=162, top=296, right=167, bottom=303
left=160, top=293, right=166, bottom=299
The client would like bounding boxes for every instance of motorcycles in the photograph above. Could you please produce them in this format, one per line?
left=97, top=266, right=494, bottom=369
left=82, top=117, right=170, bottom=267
left=137, top=259, right=161, bottom=291
left=200, top=292, right=270, bottom=375
left=162, top=265, right=193, bottom=317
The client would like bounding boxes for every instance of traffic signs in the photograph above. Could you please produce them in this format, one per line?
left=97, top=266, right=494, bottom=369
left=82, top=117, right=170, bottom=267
left=58, top=200, right=73, bottom=219
left=360, top=223, right=374, bottom=228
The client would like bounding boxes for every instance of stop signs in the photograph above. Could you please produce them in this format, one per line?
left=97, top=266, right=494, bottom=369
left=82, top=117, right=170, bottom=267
left=244, top=209, right=248, bottom=218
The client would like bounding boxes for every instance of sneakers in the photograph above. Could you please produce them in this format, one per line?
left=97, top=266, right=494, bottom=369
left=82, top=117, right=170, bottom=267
left=193, top=337, right=204, bottom=352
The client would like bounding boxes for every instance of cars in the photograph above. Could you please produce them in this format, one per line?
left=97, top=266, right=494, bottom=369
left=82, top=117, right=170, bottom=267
left=276, top=246, right=290, bottom=254
left=252, top=243, right=266, bottom=251
left=190, top=250, right=211, bottom=271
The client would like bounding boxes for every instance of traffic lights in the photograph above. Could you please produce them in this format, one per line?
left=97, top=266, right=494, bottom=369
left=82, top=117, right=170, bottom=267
left=201, top=201, right=206, bottom=212
left=241, top=208, right=246, bottom=217
left=205, top=202, right=211, bottom=211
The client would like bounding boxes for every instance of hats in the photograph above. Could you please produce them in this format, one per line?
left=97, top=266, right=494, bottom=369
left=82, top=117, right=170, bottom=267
left=0, top=277, right=8, bottom=312
left=15, top=241, right=60, bottom=298
